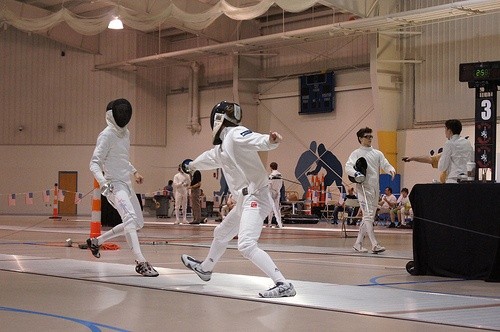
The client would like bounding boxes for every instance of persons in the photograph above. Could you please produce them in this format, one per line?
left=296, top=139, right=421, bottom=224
left=333, top=186, right=362, bottom=225
left=265, top=162, right=284, bottom=228
left=374, top=186, right=414, bottom=228
left=86, top=99, right=159, bottom=277
left=164, top=180, right=173, bottom=197
left=402, top=119, right=474, bottom=183
left=181, top=101, right=296, bottom=297
left=171, top=159, right=202, bottom=225
left=346, top=127, right=395, bottom=254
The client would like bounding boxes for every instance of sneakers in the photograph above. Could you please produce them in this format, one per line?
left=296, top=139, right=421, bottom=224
left=258, top=281, right=296, bottom=298
left=87, top=238, right=100, bottom=258
left=181, top=254, right=211, bottom=282
left=134, top=260, right=159, bottom=277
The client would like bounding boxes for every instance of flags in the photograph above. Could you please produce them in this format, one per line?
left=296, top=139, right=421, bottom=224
left=25, top=192, right=33, bottom=204
left=74, top=193, right=82, bottom=204
left=43, top=190, right=50, bottom=202
left=8, top=193, right=16, bottom=206
left=58, top=190, right=65, bottom=202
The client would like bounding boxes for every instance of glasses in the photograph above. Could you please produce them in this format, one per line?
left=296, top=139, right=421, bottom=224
left=364, top=135, right=373, bottom=139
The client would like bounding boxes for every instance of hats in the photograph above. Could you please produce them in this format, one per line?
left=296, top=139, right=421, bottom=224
left=219, top=101, right=243, bottom=126
left=105, top=101, right=113, bottom=111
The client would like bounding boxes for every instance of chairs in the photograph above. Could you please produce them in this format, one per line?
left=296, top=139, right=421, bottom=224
left=320, top=200, right=338, bottom=224
left=341, top=197, right=365, bottom=238
left=372, top=201, right=404, bottom=226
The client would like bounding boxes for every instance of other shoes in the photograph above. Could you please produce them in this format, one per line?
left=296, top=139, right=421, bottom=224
left=352, top=244, right=368, bottom=253
left=372, top=245, right=385, bottom=251
left=189, top=221, right=200, bottom=224
left=175, top=220, right=189, bottom=224
left=396, top=222, right=400, bottom=227
left=389, top=223, right=395, bottom=227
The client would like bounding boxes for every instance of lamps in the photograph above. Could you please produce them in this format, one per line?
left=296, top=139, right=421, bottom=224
left=107, top=11, right=124, bottom=30
